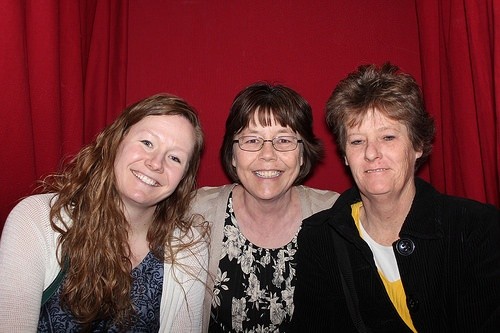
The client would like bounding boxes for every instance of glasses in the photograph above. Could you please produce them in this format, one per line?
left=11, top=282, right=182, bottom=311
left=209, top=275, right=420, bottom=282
left=233, top=135, right=303, bottom=151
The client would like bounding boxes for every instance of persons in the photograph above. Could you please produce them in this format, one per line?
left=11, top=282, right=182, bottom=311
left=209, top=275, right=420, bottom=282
left=1, top=93, right=217, bottom=333
left=184, top=79, right=341, bottom=333
left=292, top=60, right=500, bottom=333
